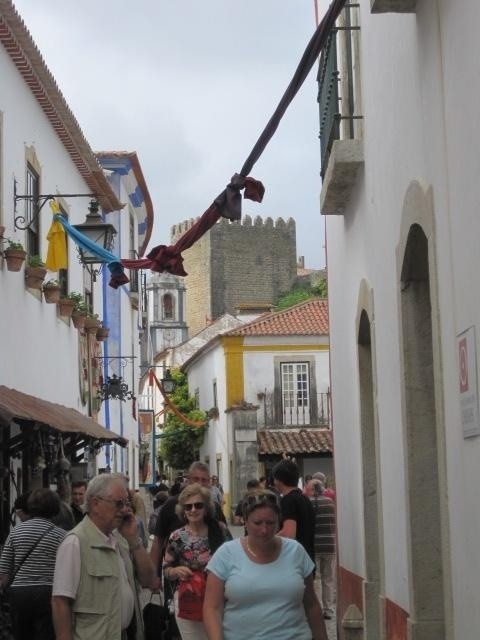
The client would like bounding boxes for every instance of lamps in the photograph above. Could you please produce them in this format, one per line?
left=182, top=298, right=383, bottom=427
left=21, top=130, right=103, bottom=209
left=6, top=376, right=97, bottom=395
left=139, top=365, right=177, bottom=394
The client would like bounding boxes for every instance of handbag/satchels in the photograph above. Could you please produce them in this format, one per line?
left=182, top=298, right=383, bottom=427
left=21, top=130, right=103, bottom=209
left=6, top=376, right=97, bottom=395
left=143, top=592, right=183, bottom=640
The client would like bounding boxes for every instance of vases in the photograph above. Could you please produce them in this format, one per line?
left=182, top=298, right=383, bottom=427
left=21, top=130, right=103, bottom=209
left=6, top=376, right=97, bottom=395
left=97, top=328, right=110, bottom=341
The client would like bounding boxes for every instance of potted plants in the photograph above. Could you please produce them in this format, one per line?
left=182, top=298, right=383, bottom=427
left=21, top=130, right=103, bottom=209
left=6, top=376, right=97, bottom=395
left=43, top=278, right=63, bottom=303
left=25, top=254, right=47, bottom=288
left=4, top=240, right=27, bottom=272
left=59, top=292, right=82, bottom=316
left=72, top=302, right=89, bottom=328
left=84, top=314, right=99, bottom=334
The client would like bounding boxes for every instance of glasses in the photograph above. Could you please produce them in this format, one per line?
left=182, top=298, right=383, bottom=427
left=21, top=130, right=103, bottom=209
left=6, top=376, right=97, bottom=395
left=181, top=502, right=204, bottom=511
left=90, top=495, right=130, bottom=508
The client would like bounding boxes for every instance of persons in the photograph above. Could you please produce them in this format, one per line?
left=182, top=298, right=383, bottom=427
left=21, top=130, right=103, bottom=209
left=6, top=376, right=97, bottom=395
left=1, top=453, right=337, bottom=640
